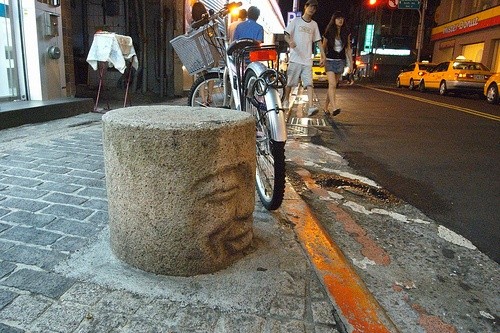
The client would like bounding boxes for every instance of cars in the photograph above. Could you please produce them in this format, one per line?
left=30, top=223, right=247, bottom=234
left=419, top=56, right=500, bottom=104
left=310, top=54, right=339, bottom=89
left=395, top=60, right=438, bottom=91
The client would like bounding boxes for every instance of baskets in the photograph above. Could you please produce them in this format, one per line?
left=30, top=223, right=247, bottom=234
left=169, top=22, right=226, bottom=76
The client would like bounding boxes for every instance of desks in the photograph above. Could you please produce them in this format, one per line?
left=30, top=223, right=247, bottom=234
left=93, top=33, right=135, bottom=116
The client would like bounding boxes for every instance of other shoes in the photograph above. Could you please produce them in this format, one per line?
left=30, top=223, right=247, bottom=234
left=305, top=0, right=320, bottom=8
left=323, top=109, right=330, bottom=116
left=283, top=100, right=289, bottom=110
left=308, top=107, right=319, bottom=116
left=332, top=108, right=341, bottom=116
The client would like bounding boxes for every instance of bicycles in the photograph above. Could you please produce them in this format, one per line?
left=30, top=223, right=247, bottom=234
left=169, top=2, right=287, bottom=212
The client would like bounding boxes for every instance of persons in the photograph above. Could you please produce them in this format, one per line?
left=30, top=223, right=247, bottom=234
left=233, top=6, right=264, bottom=66
left=283, top=0, right=326, bottom=116
left=228, top=9, right=247, bottom=43
left=320, top=12, right=354, bottom=116
